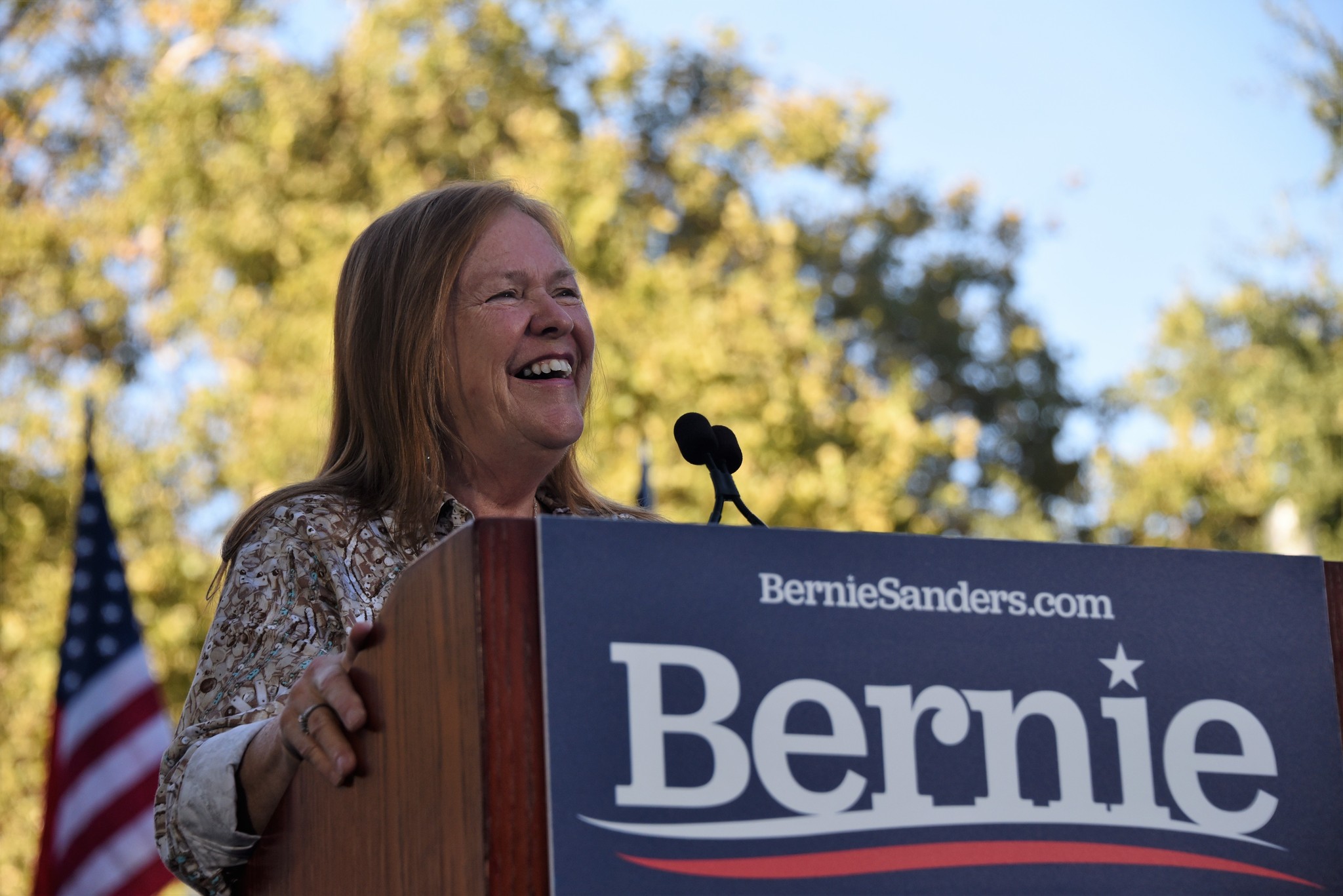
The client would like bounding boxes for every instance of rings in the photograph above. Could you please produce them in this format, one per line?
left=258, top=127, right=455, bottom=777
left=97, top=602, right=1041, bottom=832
left=298, top=702, right=328, bottom=736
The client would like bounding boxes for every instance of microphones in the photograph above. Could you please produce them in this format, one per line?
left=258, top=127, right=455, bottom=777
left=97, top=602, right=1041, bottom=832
left=674, top=412, right=768, bottom=528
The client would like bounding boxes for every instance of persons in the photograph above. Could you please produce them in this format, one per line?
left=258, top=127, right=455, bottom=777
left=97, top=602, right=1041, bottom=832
left=152, top=176, right=668, bottom=896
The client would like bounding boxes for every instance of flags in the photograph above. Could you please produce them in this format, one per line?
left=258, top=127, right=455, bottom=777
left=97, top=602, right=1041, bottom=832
left=32, top=453, right=177, bottom=896
